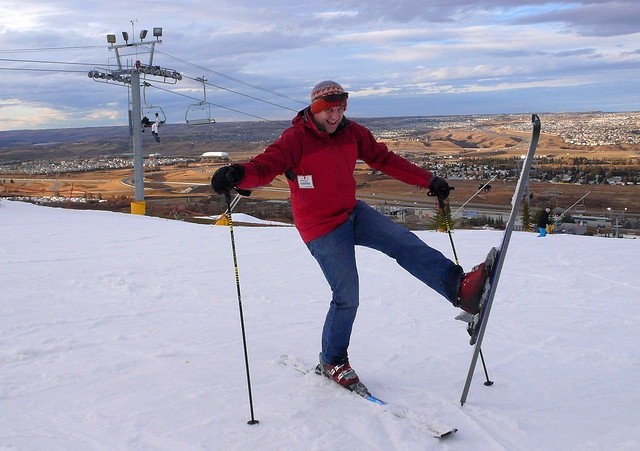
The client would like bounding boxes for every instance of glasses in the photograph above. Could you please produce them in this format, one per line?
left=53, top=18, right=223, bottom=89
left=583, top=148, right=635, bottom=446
left=319, top=92, right=349, bottom=102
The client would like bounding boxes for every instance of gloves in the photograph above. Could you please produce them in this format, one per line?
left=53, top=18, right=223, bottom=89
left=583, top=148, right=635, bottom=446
left=428, top=176, right=454, bottom=199
left=211, top=163, right=245, bottom=194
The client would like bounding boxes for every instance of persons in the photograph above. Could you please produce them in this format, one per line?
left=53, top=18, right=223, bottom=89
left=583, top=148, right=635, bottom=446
left=151, top=113, right=160, bottom=134
left=209, top=80, right=492, bottom=388
left=537, top=207, right=551, bottom=238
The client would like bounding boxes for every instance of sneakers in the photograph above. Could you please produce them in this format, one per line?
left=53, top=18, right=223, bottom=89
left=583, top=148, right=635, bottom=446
left=321, top=360, right=359, bottom=386
left=459, top=247, right=499, bottom=315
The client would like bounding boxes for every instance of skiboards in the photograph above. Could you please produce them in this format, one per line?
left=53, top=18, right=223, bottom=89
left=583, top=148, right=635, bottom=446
left=277, top=114, right=541, bottom=438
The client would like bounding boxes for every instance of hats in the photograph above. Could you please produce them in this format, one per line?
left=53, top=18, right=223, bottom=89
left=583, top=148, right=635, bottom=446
left=311, top=81, right=347, bottom=115
